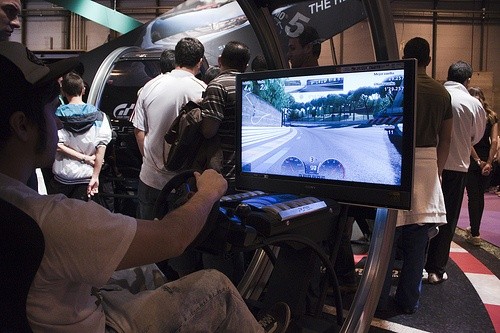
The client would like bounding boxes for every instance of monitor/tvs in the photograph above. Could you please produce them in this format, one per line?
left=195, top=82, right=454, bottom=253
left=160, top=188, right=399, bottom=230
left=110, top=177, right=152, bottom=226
left=235, top=59, right=417, bottom=212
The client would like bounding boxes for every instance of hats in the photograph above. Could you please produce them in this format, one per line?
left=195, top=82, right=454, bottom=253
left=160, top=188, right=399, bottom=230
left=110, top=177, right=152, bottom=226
left=1, top=40, right=78, bottom=98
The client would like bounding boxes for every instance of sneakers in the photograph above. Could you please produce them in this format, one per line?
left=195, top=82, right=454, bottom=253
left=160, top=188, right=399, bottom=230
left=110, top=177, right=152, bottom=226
left=259, top=302, right=291, bottom=333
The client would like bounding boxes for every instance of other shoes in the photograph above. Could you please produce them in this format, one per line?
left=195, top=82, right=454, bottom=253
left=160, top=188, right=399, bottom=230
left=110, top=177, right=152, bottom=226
left=467, top=234, right=482, bottom=245
left=466, top=226, right=472, bottom=236
left=429, top=272, right=448, bottom=284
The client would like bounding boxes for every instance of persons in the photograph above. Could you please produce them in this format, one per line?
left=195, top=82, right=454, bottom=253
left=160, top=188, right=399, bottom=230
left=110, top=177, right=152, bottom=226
left=427, top=61, right=484, bottom=284
left=0, top=0, right=21, bottom=41
left=0, top=40, right=290, bottom=333
left=379, top=37, right=452, bottom=314
left=129, top=38, right=208, bottom=279
left=53, top=72, right=112, bottom=202
left=55, top=59, right=90, bottom=105
left=287, top=24, right=359, bottom=298
left=201, top=42, right=250, bottom=287
left=465, top=86, right=499, bottom=246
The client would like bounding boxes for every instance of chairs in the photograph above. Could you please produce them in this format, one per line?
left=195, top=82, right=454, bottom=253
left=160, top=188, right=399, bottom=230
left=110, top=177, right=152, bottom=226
left=0, top=196, right=46, bottom=333
